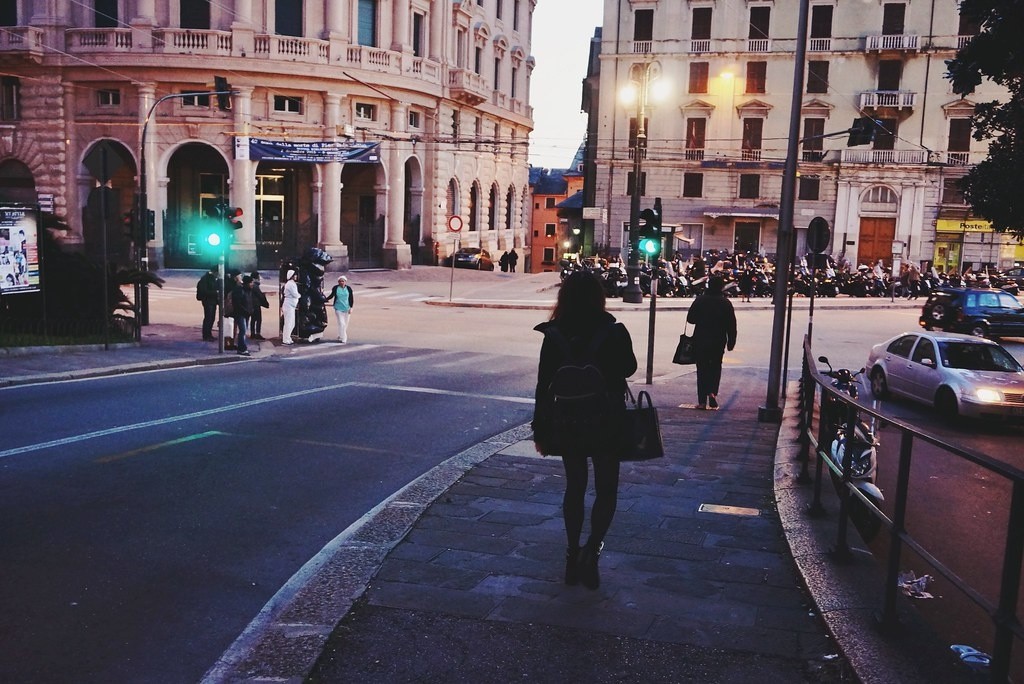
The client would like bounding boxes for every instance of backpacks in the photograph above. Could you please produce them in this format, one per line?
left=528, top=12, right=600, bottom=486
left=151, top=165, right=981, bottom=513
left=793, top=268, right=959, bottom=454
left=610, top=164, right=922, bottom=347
left=544, top=322, right=614, bottom=457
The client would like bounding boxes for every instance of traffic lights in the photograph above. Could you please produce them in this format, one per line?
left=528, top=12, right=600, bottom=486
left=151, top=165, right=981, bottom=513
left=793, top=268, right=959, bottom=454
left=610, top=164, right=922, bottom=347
left=224, top=206, right=244, bottom=244
left=122, top=211, right=136, bottom=243
left=201, top=203, right=225, bottom=259
left=639, top=208, right=661, bottom=260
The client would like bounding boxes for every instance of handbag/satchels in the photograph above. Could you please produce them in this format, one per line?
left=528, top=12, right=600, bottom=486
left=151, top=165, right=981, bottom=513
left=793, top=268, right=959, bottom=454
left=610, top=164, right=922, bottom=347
left=672, top=320, right=696, bottom=364
left=618, top=391, right=664, bottom=462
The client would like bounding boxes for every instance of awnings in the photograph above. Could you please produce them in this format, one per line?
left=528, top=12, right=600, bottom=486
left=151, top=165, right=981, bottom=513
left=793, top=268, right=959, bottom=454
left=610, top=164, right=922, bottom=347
left=703, top=207, right=779, bottom=218
left=674, top=233, right=695, bottom=245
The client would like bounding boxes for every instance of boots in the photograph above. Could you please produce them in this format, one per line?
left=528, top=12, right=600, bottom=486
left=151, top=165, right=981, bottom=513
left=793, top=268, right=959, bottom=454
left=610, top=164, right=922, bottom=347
left=576, top=536, right=602, bottom=574
left=566, top=548, right=580, bottom=584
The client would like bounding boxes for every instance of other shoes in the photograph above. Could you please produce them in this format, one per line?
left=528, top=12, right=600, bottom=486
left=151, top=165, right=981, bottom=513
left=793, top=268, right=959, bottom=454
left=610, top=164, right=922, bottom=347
left=283, top=340, right=294, bottom=345
left=255, top=334, right=265, bottom=340
left=696, top=404, right=705, bottom=409
left=211, top=336, right=217, bottom=340
left=237, top=350, right=248, bottom=354
left=203, top=337, right=214, bottom=342
left=710, top=393, right=718, bottom=407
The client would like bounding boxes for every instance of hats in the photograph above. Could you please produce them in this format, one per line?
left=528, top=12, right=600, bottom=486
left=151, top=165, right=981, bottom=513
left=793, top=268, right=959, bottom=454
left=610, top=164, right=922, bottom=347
left=338, top=275, right=347, bottom=282
left=287, top=269, right=295, bottom=279
left=242, top=275, right=253, bottom=283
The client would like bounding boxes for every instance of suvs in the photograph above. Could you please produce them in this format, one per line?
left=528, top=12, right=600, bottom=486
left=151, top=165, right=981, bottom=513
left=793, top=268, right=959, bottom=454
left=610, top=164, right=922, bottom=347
left=918, top=284, right=1024, bottom=338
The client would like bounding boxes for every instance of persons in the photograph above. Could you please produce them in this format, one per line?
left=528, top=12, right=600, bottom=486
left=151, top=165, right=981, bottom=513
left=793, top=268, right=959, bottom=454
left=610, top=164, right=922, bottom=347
left=229, top=270, right=242, bottom=348
left=908, top=263, right=921, bottom=300
left=282, top=271, right=301, bottom=346
left=326, top=276, right=355, bottom=344
left=741, top=263, right=756, bottom=302
left=232, top=275, right=254, bottom=355
left=250, top=272, right=270, bottom=341
left=687, top=277, right=737, bottom=410
left=873, top=261, right=885, bottom=297
left=482, top=249, right=491, bottom=259
left=500, top=249, right=519, bottom=273
left=532, top=273, right=638, bottom=591
left=201, top=265, right=218, bottom=341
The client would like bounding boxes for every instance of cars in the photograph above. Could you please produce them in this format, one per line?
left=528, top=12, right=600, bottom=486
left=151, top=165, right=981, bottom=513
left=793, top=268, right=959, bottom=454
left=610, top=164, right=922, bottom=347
left=445, top=246, right=495, bottom=271
left=996, top=266, right=1024, bottom=291
left=866, top=331, right=1024, bottom=424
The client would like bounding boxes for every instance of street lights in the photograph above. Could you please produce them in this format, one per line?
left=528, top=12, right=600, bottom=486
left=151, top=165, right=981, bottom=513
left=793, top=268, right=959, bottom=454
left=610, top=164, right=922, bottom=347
left=139, top=86, right=241, bottom=326
left=622, top=62, right=666, bottom=303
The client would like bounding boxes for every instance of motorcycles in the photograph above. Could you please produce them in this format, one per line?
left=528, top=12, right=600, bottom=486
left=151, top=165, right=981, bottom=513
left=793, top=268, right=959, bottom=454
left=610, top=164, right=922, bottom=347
left=828, top=413, right=888, bottom=544
left=818, top=356, right=866, bottom=441
left=555, top=248, right=1021, bottom=297
